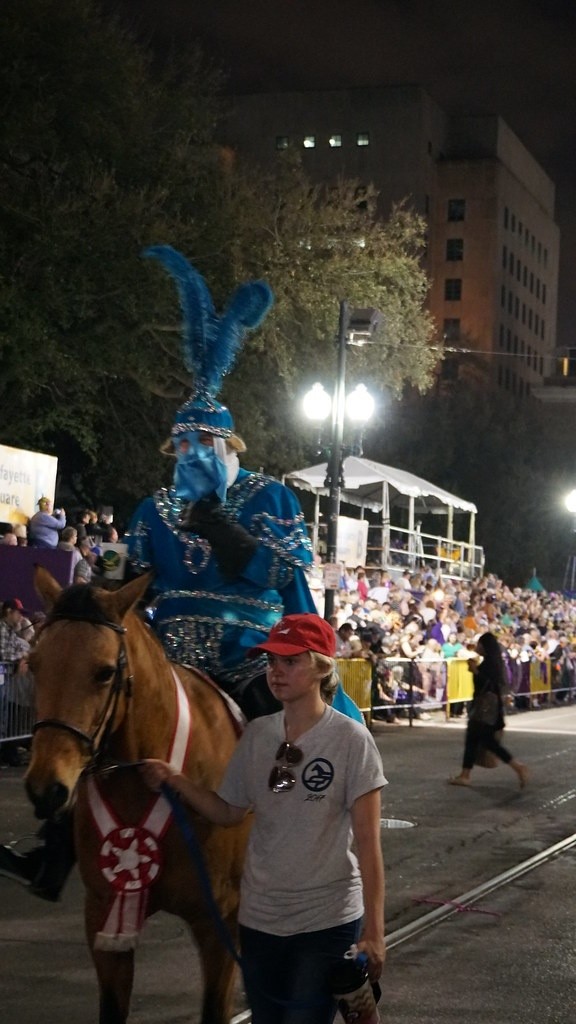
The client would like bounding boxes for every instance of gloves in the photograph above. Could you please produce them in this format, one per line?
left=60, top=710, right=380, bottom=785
left=182, top=498, right=251, bottom=579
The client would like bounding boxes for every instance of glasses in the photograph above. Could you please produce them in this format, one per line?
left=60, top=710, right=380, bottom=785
left=267, top=742, right=304, bottom=794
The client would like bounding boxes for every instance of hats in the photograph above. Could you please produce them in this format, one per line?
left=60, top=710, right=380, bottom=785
left=3, top=597, right=29, bottom=612
left=247, top=612, right=338, bottom=659
left=90, top=546, right=101, bottom=554
left=142, top=244, right=273, bottom=457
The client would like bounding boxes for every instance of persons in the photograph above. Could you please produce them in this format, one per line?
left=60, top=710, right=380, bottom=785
left=122, top=394, right=365, bottom=727
left=447, top=632, right=528, bottom=789
left=329, top=520, right=576, bottom=723
left=0, top=493, right=128, bottom=585
left=0, top=598, right=46, bottom=766
left=135, top=614, right=389, bottom=1024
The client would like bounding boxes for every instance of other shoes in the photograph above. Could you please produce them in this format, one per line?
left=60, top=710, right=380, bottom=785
left=387, top=716, right=400, bottom=724
left=0, top=835, right=66, bottom=901
left=514, top=763, right=528, bottom=788
left=447, top=774, right=471, bottom=787
left=419, top=712, right=432, bottom=720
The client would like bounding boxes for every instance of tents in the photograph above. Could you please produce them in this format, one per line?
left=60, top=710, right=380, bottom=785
left=281, top=456, right=477, bottom=577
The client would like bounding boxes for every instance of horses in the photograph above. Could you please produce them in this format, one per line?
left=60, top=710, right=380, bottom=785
left=23, top=568, right=252, bottom=1024
